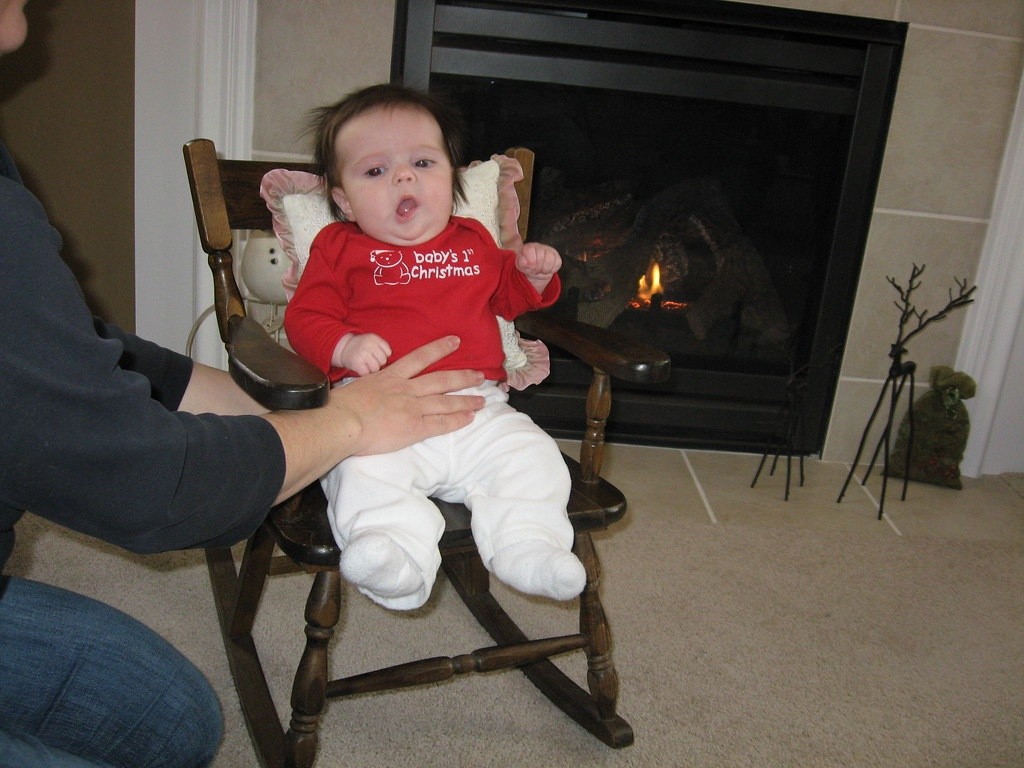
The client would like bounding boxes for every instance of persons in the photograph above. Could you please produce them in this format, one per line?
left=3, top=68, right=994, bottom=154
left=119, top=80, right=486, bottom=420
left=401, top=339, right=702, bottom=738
left=0, top=0, right=485, bottom=768
left=284, top=84, right=587, bottom=611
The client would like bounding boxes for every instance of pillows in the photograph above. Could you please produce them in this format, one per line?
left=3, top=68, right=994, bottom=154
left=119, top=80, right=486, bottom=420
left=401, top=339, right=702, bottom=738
left=259, top=152, right=550, bottom=391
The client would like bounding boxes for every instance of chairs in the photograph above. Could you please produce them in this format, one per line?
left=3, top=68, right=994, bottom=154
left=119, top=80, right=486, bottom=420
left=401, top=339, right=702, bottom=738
left=182, top=136, right=672, bottom=768
